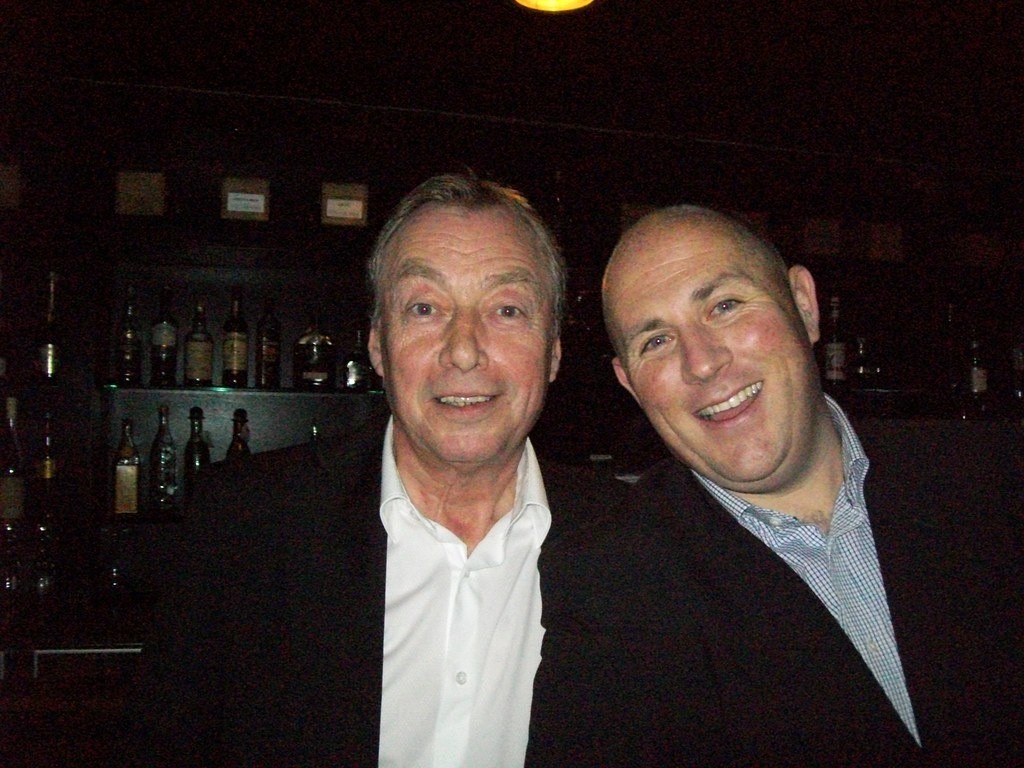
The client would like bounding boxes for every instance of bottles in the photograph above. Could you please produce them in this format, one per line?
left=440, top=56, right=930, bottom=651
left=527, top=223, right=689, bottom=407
left=42, top=413, right=56, bottom=488
left=965, top=337, right=987, bottom=392
left=183, top=304, right=215, bottom=386
left=183, top=408, right=210, bottom=479
left=0, top=398, right=25, bottom=540
left=117, top=306, right=142, bottom=382
left=150, top=305, right=178, bottom=385
left=847, top=338, right=881, bottom=389
left=149, top=407, right=175, bottom=512
left=221, top=299, right=248, bottom=387
left=227, top=408, right=250, bottom=458
left=339, top=330, right=375, bottom=391
left=819, top=295, right=849, bottom=389
left=36, top=272, right=65, bottom=382
left=256, top=313, right=281, bottom=388
left=293, top=315, right=336, bottom=385
left=310, top=427, right=319, bottom=441
left=113, top=420, right=142, bottom=517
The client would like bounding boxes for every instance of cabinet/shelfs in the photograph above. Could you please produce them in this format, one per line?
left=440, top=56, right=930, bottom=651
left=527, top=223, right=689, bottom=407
left=0, top=76, right=1024, bottom=651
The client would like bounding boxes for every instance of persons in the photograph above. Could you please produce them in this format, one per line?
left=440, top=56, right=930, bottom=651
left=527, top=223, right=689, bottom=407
left=599, top=204, right=1024, bottom=768
left=117, top=172, right=734, bottom=768
